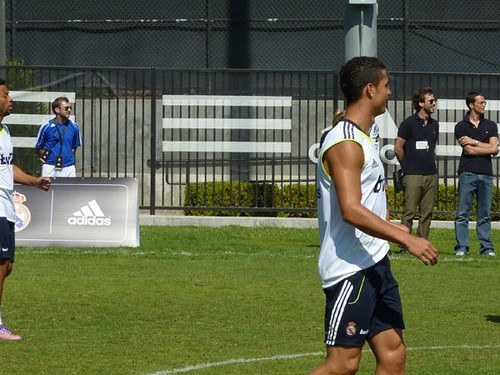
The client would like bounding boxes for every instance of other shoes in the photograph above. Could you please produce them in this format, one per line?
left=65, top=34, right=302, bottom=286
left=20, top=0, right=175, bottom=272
left=0, top=324, right=22, bottom=340
left=455, top=250, right=469, bottom=256
left=481, top=251, right=496, bottom=255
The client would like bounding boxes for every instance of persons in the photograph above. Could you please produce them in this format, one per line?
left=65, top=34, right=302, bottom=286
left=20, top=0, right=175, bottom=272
left=453, top=93, right=500, bottom=257
left=306, top=55, right=438, bottom=374
left=35, top=97, right=82, bottom=178
left=395, top=88, right=440, bottom=253
left=0, top=85, right=51, bottom=341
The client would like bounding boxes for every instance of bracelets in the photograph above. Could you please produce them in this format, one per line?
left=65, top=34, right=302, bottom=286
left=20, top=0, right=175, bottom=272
left=475, top=140, right=478, bottom=144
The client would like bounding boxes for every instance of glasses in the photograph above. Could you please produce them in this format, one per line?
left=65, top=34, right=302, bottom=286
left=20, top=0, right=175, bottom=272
left=427, top=98, right=437, bottom=104
left=477, top=101, right=487, bottom=105
left=63, top=106, right=72, bottom=111
